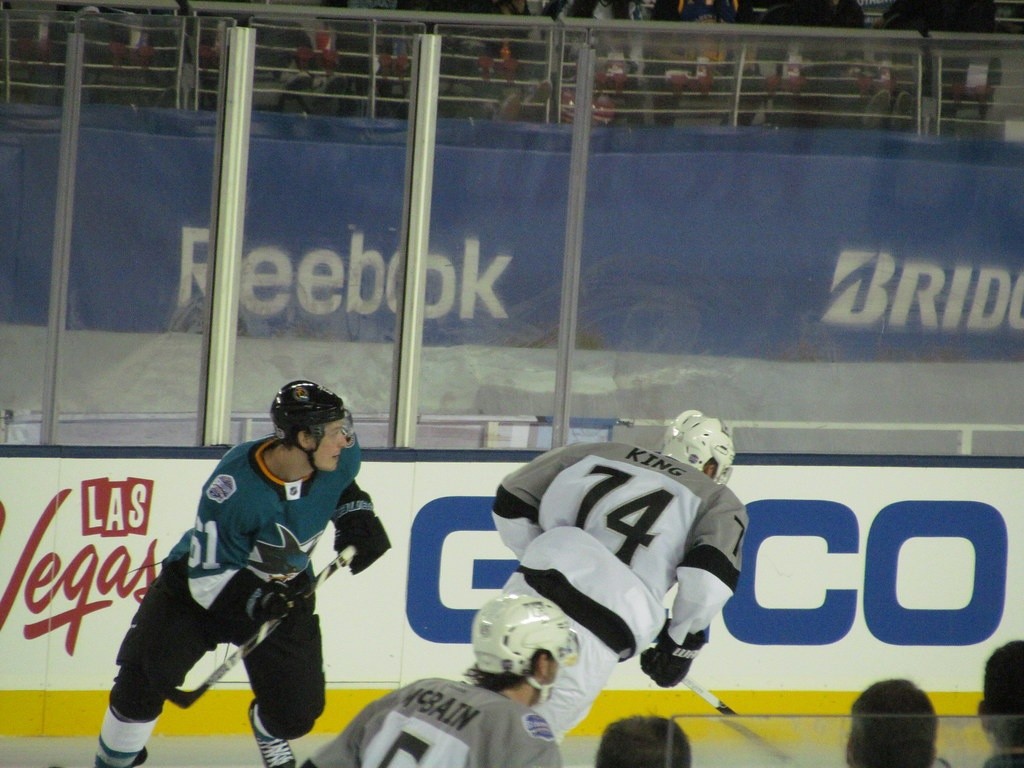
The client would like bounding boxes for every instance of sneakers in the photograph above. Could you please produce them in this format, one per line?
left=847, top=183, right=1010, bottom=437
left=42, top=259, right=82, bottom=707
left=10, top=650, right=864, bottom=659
left=248, top=699, right=296, bottom=768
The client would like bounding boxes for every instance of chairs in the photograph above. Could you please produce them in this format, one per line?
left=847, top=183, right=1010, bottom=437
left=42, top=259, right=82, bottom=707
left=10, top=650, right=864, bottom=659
left=0, top=0, right=1004, bottom=143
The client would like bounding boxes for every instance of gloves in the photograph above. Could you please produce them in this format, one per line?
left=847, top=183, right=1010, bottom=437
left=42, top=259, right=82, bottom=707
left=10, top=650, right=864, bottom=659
left=639, top=618, right=706, bottom=689
left=333, top=491, right=392, bottom=576
left=207, top=566, right=314, bottom=640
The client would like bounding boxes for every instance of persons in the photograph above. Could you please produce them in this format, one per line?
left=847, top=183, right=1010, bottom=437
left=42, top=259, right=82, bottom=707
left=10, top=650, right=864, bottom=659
left=93, top=381, right=391, bottom=768
left=490, top=411, right=750, bottom=746
left=847, top=679, right=937, bottom=768
left=977, top=641, right=1024, bottom=768
left=0, top=0, right=1001, bottom=136
left=595, top=717, right=692, bottom=768
left=300, top=594, right=571, bottom=768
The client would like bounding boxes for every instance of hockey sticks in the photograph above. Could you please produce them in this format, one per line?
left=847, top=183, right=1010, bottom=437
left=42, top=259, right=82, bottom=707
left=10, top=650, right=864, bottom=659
left=143, top=544, right=358, bottom=708
left=681, top=677, right=737, bottom=714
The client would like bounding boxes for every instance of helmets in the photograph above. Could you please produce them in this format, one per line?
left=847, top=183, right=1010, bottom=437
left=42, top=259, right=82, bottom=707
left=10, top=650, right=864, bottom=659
left=270, top=380, right=345, bottom=444
left=661, top=409, right=735, bottom=483
left=471, top=594, right=582, bottom=676
